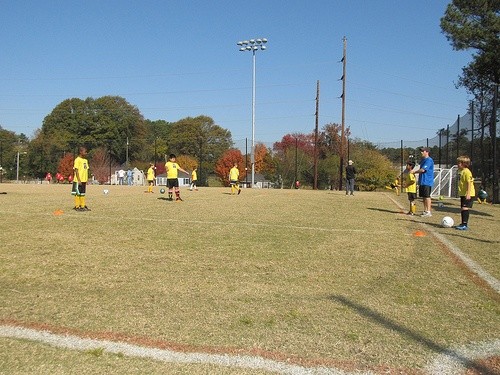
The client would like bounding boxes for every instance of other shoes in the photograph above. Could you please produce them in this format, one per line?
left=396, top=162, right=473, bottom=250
left=238, top=189, right=241, bottom=195
left=420, top=210, right=432, bottom=217
left=74, top=204, right=91, bottom=212
left=456, top=225, right=467, bottom=230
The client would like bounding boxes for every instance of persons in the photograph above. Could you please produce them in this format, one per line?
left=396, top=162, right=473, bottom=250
left=132, top=166, right=145, bottom=186
left=295, top=180, right=301, bottom=189
left=385, top=176, right=401, bottom=196
left=117, top=169, right=126, bottom=185
left=146, top=162, right=156, bottom=193
left=56, top=172, right=66, bottom=184
left=46, top=172, right=52, bottom=184
left=404, top=147, right=434, bottom=217
left=126, top=167, right=132, bottom=185
left=346, top=160, right=357, bottom=196
left=456, top=156, right=475, bottom=230
left=164, top=153, right=189, bottom=201
left=189, top=166, right=199, bottom=191
left=476, top=186, right=492, bottom=206
left=67, top=173, right=73, bottom=184
left=229, top=162, right=241, bottom=195
left=71, top=147, right=91, bottom=212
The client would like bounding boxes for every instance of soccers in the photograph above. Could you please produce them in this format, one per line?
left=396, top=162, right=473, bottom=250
left=160, top=189, right=165, bottom=194
left=439, top=194, right=445, bottom=200
left=441, top=216, right=454, bottom=228
left=438, top=203, right=444, bottom=209
left=104, top=189, right=109, bottom=195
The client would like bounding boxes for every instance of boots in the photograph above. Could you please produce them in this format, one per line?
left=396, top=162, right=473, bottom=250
left=175, top=190, right=184, bottom=202
left=169, top=191, right=173, bottom=201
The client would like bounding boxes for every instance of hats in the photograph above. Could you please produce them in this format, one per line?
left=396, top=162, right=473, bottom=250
left=419, top=147, right=431, bottom=152
left=348, top=160, right=354, bottom=165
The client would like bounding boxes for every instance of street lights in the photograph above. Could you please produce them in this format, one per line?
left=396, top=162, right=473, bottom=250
left=236, top=38, right=268, bottom=188
left=17, top=151, right=27, bottom=183
